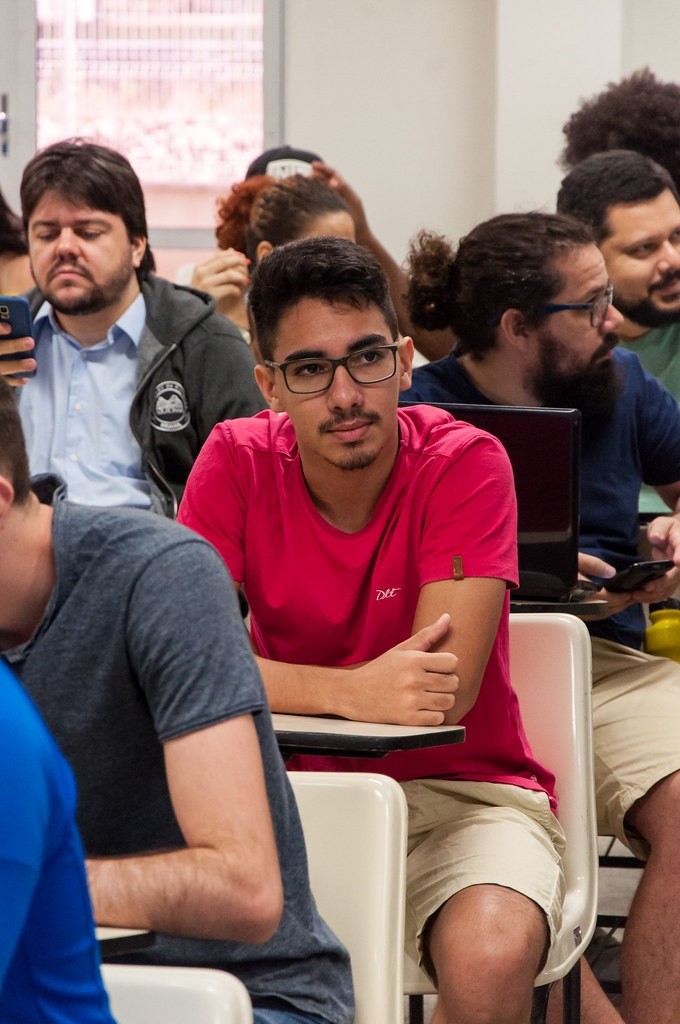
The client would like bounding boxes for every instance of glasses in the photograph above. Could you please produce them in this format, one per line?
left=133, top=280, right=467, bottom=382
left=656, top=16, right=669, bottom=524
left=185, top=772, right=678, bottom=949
left=521, top=283, right=614, bottom=327
left=262, top=342, right=400, bottom=394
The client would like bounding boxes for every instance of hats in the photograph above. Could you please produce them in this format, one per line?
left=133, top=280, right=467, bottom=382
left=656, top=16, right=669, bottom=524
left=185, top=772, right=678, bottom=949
left=245, top=146, right=325, bottom=181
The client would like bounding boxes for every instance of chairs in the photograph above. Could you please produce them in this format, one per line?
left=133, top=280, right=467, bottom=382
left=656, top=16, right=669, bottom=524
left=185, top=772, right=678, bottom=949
left=287, top=772, right=408, bottom=1024
left=403, top=612, right=594, bottom=993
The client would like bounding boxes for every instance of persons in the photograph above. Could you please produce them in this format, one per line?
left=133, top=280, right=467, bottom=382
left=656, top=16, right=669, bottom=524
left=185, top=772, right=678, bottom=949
left=0, top=66, right=680, bottom=520
left=401, top=211, right=680, bottom=1024
left=0, top=658, right=119, bottom=1024
left=177, top=237, right=566, bottom=1024
left=0, top=377, right=356, bottom=1024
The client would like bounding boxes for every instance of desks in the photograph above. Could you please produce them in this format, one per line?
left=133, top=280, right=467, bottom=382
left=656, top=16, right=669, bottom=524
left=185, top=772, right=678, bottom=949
left=271, top=712, right=464, bottom=755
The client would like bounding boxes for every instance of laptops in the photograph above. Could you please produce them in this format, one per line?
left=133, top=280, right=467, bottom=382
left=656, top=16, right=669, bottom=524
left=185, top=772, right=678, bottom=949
left=395, top=400, right=602, bottom=605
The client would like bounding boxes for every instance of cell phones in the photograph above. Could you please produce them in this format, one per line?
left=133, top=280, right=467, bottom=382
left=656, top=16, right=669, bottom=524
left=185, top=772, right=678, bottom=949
left=598, top=560, right=675, bottom=593
left=0, top=295, right=37, bottom=379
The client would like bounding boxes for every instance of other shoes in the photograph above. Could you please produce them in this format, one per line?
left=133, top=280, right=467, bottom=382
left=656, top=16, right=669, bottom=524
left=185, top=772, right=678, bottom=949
left=584, top=924, right=623, bottom=1005
